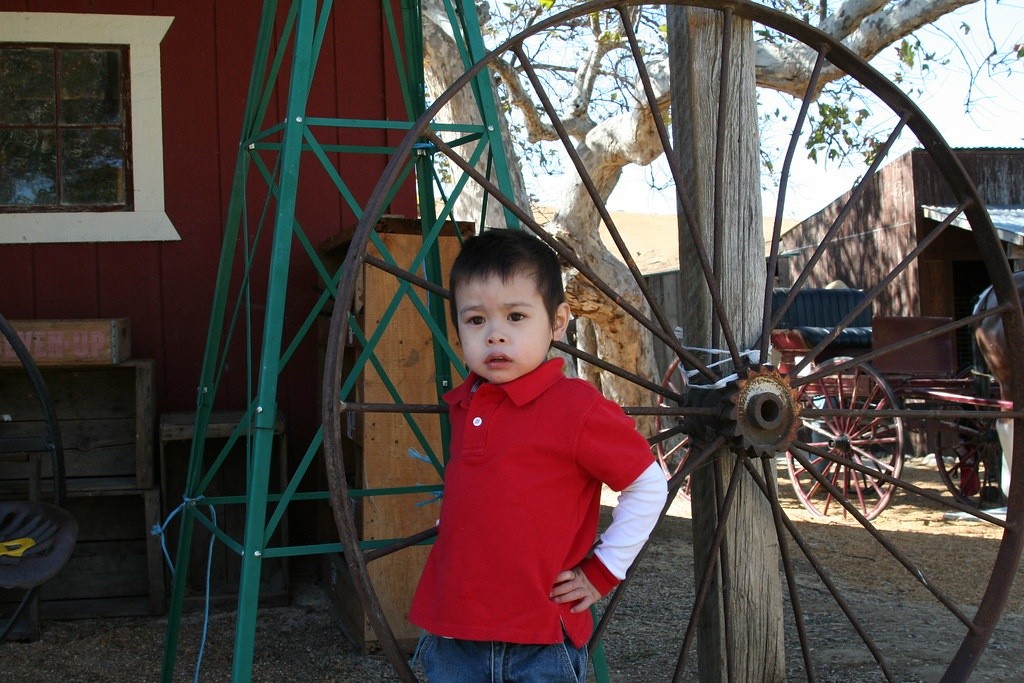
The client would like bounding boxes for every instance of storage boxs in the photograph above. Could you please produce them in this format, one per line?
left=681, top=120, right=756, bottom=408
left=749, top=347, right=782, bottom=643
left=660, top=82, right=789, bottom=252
left=872, top=316, right=957, bottom=374
left=0, top=317, right=133, bottom=365
left=0, top=360, right=156, bottom=494
left=160, top=412, right=297, bottom=614
left=0, top=491, right=165, bottom=621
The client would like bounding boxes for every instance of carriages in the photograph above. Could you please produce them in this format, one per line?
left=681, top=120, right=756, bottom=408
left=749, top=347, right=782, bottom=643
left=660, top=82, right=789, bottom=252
left=757, top=267, right=1024, bottom=528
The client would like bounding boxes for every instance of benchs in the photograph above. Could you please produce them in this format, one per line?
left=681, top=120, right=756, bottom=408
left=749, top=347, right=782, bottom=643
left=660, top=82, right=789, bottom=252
left=771, top=288, right=872, bottom=348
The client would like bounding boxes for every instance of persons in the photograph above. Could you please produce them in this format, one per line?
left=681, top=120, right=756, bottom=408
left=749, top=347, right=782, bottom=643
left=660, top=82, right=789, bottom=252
left=407, top=230, right=668, bottom=683
